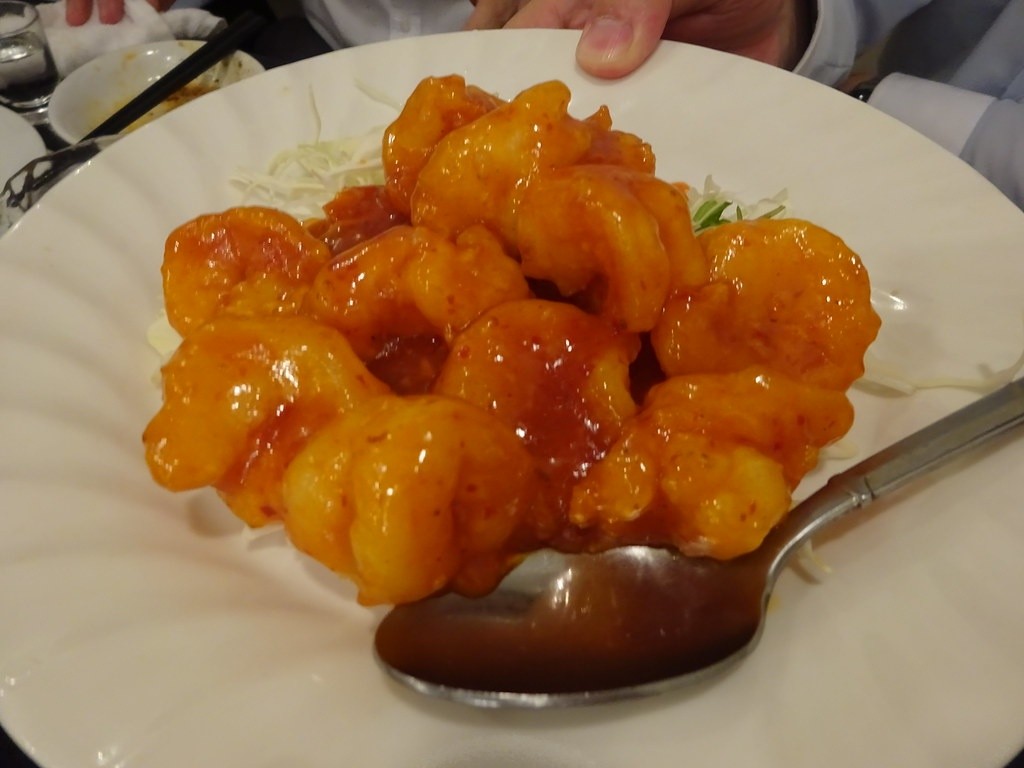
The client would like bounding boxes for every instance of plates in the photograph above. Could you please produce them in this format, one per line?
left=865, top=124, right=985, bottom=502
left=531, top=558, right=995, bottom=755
left=0, top=26, right=1024, bottom=768
left=49, top=40, right=267, bottom=151
left=0, top=104, right=52, bottom=238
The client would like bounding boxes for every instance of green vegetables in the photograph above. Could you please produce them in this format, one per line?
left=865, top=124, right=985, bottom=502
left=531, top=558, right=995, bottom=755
left=214, top=79, right=1024, bottom=583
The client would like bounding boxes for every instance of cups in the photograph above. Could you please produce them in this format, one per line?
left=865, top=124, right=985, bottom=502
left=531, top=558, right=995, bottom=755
left=0, top=0, right=62, bottom=115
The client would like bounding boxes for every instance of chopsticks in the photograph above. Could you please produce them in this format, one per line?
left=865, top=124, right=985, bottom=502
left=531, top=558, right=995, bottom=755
left=11, top=6, right=276, bottom=202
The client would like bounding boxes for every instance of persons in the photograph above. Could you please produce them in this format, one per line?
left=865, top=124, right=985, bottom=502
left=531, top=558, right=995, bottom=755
left=0, top=0, right=1024, bottom=213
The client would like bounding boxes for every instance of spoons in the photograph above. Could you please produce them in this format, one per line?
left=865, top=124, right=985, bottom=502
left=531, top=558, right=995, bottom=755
left=373, top=375, right=1024, bottom=706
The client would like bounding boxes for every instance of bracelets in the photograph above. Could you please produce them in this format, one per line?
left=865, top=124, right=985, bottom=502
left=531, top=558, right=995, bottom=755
left=849, top=73, right=886, bottom=103
